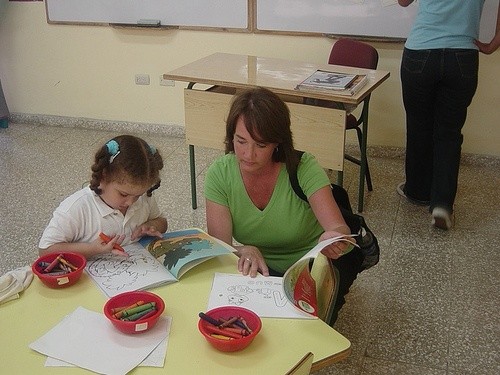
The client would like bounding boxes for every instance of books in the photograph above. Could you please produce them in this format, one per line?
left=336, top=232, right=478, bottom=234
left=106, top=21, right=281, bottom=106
left=87, top=228, right=235, bottom=298
left=209, top=234, right=361, bottom=320
left=294, top=69, right=368, bottom=97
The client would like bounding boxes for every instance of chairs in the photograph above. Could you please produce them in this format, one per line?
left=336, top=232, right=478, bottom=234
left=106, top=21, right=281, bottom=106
left=311, top=252, right=340, bottom=325
left=328, top=39, right=379, bottom=212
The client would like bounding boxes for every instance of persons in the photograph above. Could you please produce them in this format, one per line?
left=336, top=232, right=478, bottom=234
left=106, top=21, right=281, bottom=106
left=39, top=135, right=167, bottom=259
left=202, top=87, right=364, bottom=326
left=397, top=0, right=500, bottom=228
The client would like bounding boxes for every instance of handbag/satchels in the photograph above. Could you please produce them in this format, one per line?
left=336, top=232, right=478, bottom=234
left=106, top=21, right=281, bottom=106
left=288, top=148, right=380, bottom=274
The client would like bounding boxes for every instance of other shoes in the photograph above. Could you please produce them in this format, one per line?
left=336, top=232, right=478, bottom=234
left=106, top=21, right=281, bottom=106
left=432, top=207, right=451, bottom=229
left=398, top=182, right=431, bottom=210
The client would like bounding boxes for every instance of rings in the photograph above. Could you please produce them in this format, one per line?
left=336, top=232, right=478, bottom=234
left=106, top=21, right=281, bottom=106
left=246, top=258, right=251, bottom=263
left=338, top=252, right=345, bottom=256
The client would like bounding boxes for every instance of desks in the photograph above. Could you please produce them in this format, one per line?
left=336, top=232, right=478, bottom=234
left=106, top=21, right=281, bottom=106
left=164, top=52, right=390, bottom=210
left=0, top=252, right=352, bottom=375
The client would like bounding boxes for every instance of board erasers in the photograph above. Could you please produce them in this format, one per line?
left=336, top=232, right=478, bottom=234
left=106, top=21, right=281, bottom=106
left=137, top=19, right=161, bottom=25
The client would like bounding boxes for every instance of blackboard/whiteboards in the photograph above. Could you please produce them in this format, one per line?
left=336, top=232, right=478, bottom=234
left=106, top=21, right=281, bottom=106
left=44, top=0, right=251, bottom=35
left=254, top=0, right=500, bottom=43
